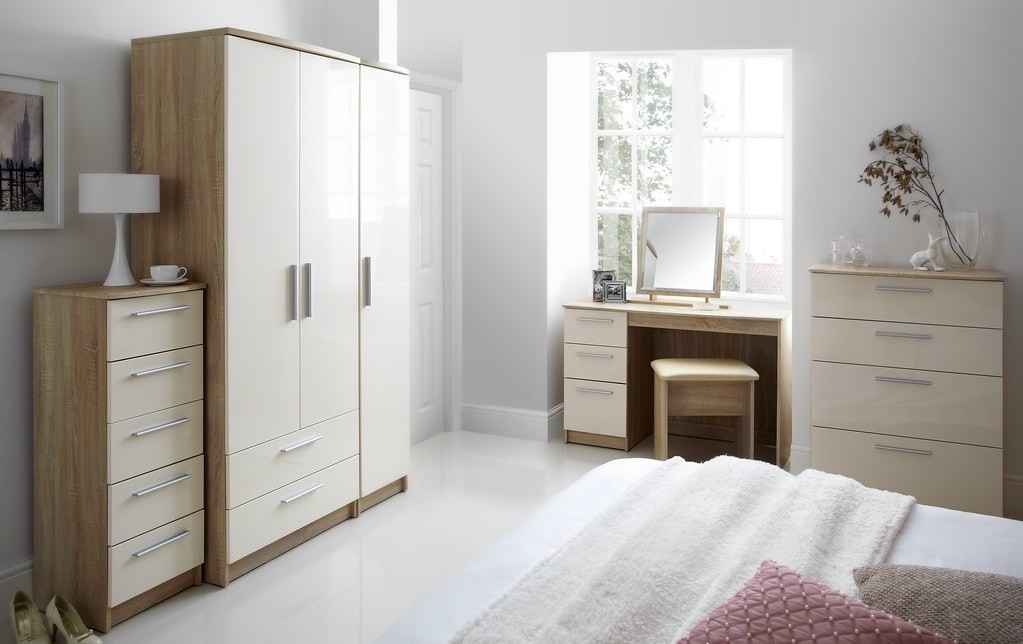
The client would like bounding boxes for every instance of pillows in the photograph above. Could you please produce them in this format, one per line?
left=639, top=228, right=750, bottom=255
left=852, top=563, right=1023, bottom=644
left=674, top=559, right=958, bottom=644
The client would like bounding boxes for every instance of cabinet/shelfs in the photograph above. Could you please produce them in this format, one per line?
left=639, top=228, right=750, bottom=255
left=358, top=56, right=411, bottom=514
left=30, top=280, right=208, bottom=636
left=809, top=260, right=1007, bottom=520
left=126, top=25, right=360, bottom=589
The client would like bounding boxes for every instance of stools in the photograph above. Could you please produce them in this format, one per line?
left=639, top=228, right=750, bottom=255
left=650, top=357, right=761, bottom=461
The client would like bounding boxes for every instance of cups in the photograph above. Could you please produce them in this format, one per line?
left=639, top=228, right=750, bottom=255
left=833, top=240, right=856, bottom=269
left=850, top=246, right=872, bottom=269
left=150, top=264, right=187, bottom=282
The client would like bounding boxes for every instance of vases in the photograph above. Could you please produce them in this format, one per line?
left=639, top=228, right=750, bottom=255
left=935, top=210, right=983, bottom=274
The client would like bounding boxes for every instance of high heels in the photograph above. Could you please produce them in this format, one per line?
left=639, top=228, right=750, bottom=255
left=45, top=594, right=103, bottom=644
left=8, top=591, right=52, bottom=644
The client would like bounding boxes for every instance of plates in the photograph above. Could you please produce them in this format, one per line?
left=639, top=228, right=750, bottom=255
left=140, top=277, right=189, bottom=286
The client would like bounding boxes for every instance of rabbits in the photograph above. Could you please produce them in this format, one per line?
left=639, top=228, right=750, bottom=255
left=908, top=232, right=946, bottom=271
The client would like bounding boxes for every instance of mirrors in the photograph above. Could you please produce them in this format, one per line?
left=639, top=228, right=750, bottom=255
left=636, top=205, right=726, bottom=299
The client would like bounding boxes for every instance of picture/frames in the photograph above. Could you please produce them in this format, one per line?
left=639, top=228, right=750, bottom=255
left=0, top=73, right=65, bottom=230
left=593, top=269, right=616, bottom=302
left=602, top=280, right=627, bottom=304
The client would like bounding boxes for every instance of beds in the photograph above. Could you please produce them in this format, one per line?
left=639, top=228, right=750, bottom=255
left=368, top=456, right=1023, bottom=644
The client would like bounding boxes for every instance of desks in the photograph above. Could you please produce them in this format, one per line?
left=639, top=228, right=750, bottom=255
left=560, top=298, right=792, bottom=468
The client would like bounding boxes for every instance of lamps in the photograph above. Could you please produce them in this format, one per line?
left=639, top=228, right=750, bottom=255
left=77, top=173, right=161, bottom=287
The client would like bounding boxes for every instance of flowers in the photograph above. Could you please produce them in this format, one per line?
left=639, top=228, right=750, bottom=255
left=858, top=122, right=973, bottom=265
